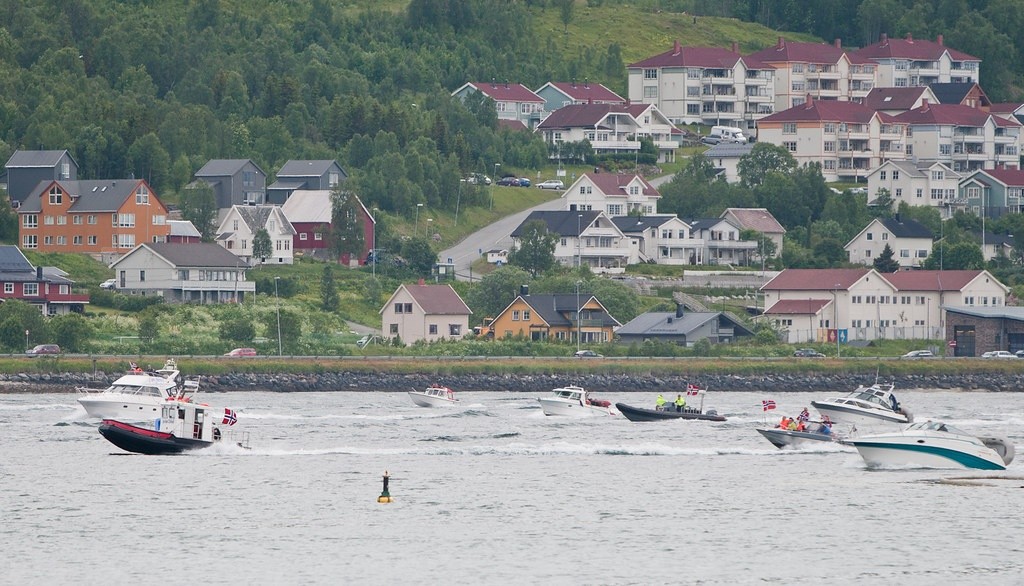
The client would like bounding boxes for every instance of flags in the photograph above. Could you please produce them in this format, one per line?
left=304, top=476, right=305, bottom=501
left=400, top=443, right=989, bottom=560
left=763, top=400, right=777, bottom=411
left=949, top=340, right=957, bottom=346
left=222, top=408, right=237, bottom=426
left=687, top=384, right=700, bottom=396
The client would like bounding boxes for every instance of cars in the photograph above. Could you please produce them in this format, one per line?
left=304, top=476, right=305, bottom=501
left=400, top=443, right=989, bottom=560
left=980, top=350, right=1024, bottom=360
left=495, top=177, right=521, bottom=187
left=520, top=178, right=531, bottom=187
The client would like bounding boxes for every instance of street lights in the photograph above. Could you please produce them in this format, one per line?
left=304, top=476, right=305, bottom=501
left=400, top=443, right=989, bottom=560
left=578, top=214, right=584, bottom=268
left=415, top=203, right=434, bottom=239
left=273, top=276, right=282, bottom=355
left=489, top=163, right=501, bottom=211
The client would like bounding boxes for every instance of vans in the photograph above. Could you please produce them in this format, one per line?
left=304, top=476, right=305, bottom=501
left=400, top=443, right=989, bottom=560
left=224, top=348, right=256, bottom=357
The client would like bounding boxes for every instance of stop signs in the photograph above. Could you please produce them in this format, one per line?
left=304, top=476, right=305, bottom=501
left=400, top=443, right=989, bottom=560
left=948, top=341, right=957, bottom=348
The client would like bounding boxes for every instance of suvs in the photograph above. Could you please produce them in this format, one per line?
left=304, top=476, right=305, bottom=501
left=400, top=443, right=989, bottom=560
left=459, top=172, right=492, bottom=186
left=900, top=350, right=934, bottom=360
left=99, top=279, right=116, bottom=290
left=793, top=348, right=826, bottom=357
left=356, top=335, right=382, bottom=346
left=364, top=248, right=409, bottom=270
left=535, top=179, right=564, bottom=190
left=24, top=344, right=61, bottom=358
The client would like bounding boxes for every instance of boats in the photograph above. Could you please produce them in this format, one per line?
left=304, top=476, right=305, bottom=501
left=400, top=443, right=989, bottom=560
left=98, top=396, right=237, bottom=455
left=613, top=381, right=728, bottom=423
left=76, top=356, right=201, bottom=421
left=811, top=383, right=913, bottom=429
left=842, top=418, right=1015, bottom=471
left=408, top=385, right=462, bottom=408
left=535, top=385, right=611, bottom=419
left=756, top=413, right=854, bottom=450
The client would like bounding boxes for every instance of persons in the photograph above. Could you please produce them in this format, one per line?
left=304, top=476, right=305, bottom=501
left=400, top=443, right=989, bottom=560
left=657, top=395, right=665, bottom=407
left=797, top=407, right=809, bottom=421
left=779, top=418, right=805, bottom=432
left=132, top=364, right=142, bottom=370
left=822, top=415, right=832, bottom=427
left=674, top=394, right=685, bottom=412
left=146, top=364, right=155, bottom=375
